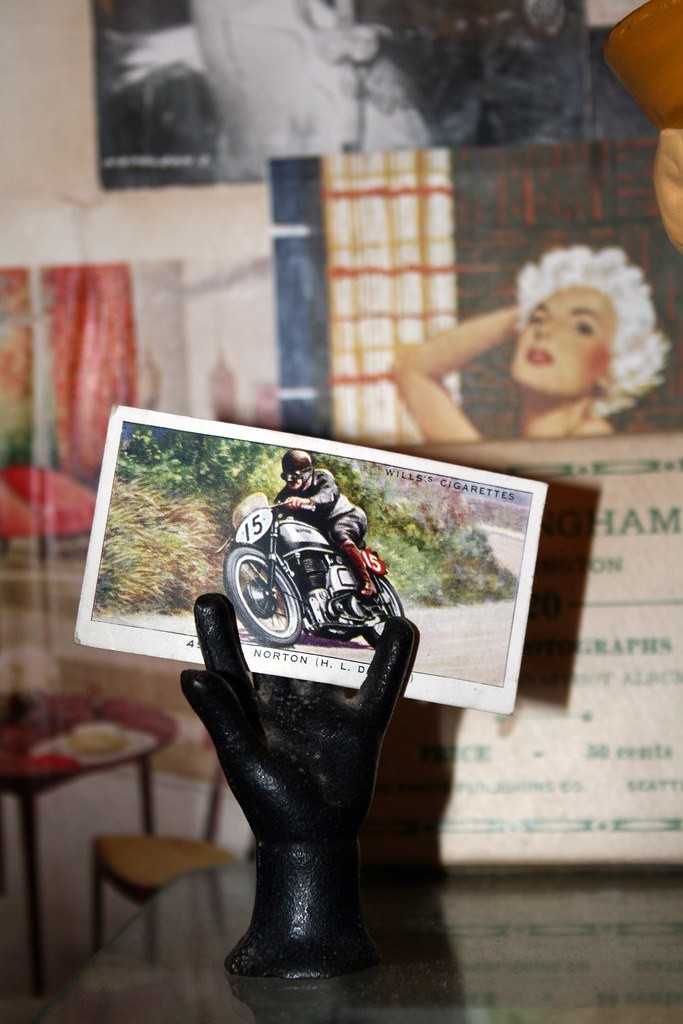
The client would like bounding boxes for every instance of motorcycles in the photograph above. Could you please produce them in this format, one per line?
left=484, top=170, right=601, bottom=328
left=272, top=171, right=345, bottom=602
left=214, top=493, right=405, bottom=648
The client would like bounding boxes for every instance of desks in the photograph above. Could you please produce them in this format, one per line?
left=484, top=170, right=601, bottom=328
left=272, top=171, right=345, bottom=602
left=28, top=865, right=683, bottom=1024
left=0, top=690, right=182, bottom=998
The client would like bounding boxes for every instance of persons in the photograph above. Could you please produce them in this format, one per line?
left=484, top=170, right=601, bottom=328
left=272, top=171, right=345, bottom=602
left=177, top=591, right=418, bottom=980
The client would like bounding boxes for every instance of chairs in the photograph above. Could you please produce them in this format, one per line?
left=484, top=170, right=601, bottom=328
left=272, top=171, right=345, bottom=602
left=91, top=761, right=257, bottom=968
